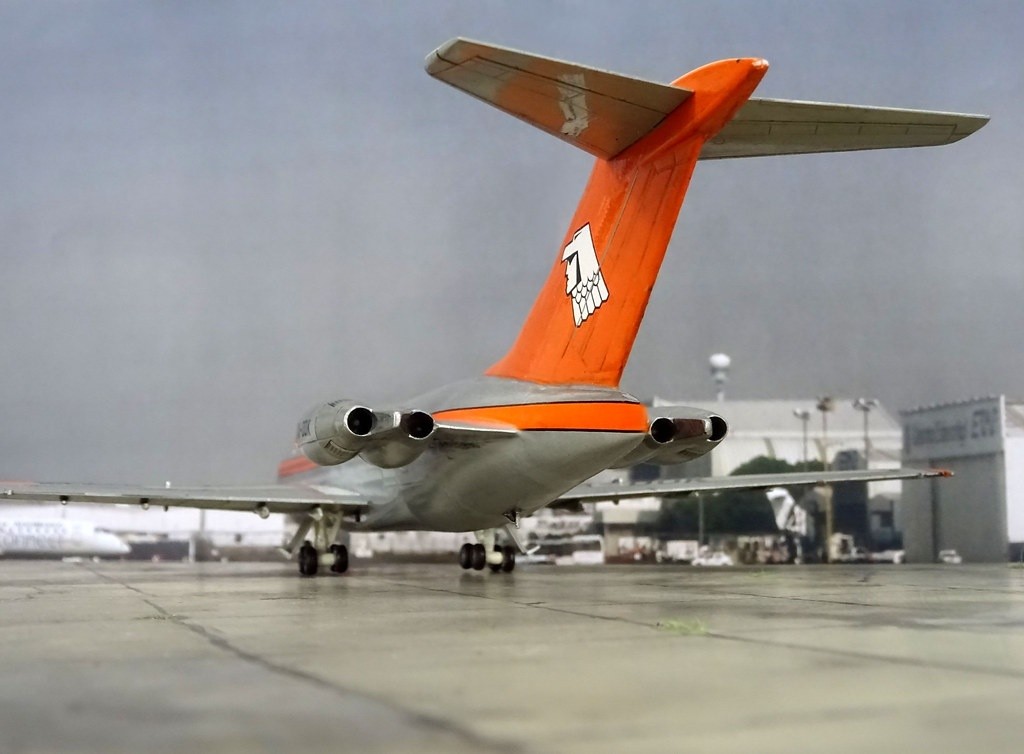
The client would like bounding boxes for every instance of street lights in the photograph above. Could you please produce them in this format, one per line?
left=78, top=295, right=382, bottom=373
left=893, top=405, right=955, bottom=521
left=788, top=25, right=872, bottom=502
left=816, top=394, right=834, bottom=558
left=853, top=397, right=879, bottom=558
left=793, top=407, right=811, bottom=506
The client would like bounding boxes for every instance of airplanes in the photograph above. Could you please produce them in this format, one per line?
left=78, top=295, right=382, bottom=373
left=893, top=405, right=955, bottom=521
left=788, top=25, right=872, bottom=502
left=0, top=36, right=991, bottom=582
